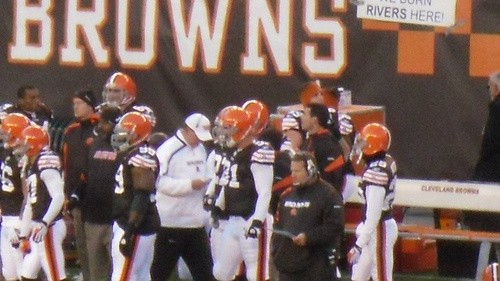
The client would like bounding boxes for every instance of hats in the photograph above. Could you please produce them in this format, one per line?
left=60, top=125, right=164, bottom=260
left=184, top=112, right=213, bottom=142
left=73, top=88, right=96, bottom=108
left=100, top=105, right=122, bottom=122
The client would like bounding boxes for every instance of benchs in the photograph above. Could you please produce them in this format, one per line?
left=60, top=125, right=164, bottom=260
left=342, top=177, right=500, bottom=281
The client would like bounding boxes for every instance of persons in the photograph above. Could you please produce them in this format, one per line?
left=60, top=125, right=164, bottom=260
left=150, top=113, right=217, bottom=281
left=203, top=99, right=275, bottom=281
left=63, top=73, right=169, bottom=281
left=0, top=85, right=67, bottom=281
left=270, top=151, right=347, bottom=281
left=470, top=72, right=500, bottom=185
left=348, top=123, right=399, bottom=281
left=269, top=79, right=354, bottom=217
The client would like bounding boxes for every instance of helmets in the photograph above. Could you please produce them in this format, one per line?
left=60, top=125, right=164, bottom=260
left=109, top=72, right=138, bottom=96
left=111, top=112, right=156, bottom=153
left=358, top=123, right=391, bottom=154
left=281, top=111, right=308, bottom=132
left=212, top=98, right=269, bottom=148
left=1, top=113, right=49, bottom=157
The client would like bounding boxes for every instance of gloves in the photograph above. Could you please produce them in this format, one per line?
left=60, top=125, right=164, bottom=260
left=347, top=245, right=362, bottom=265
left=10, top=227, right=21, bottom=249
left=245, top=220, right=264, bottom=240
left=339, top=115, right=353, bottom=135
left=119, top=228, right=134, bottom=258
left=21, top=237, right=30, bottom=255
left=32, top=222, right=48, bottom=243
left=202, top=194, right=215, bottom=212
left=66, top=192, right=80, bottom=212
